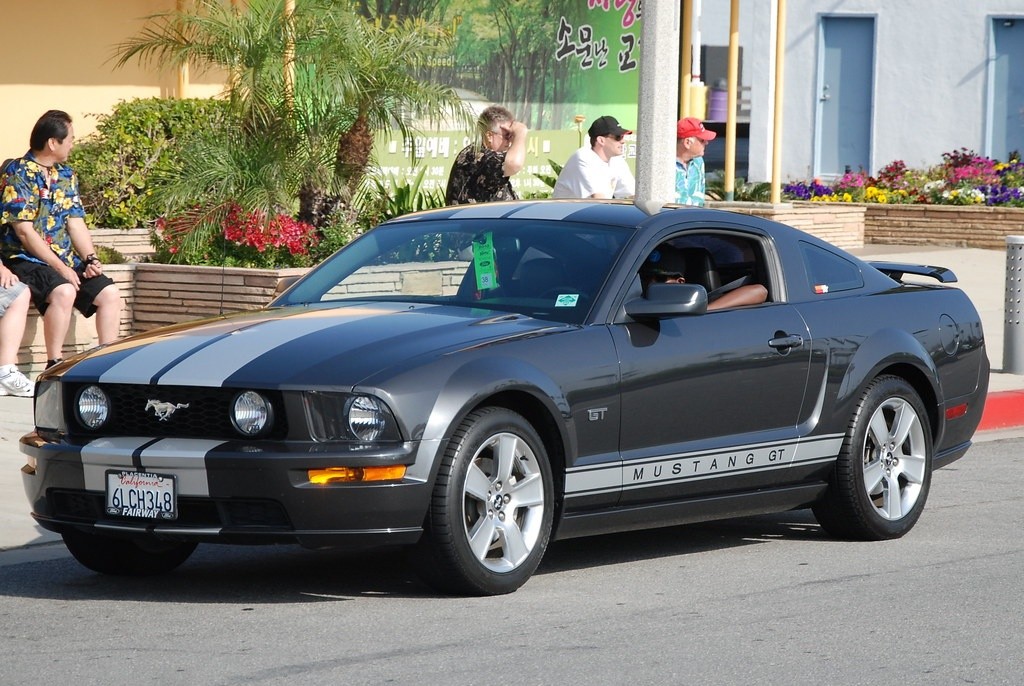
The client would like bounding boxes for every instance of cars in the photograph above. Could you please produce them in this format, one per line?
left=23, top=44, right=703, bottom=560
left=20, top=202, right=992, bottom=596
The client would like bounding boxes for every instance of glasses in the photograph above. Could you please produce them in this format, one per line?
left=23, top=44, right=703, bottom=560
left=642, top=273, right=677, bottom=284
left=488, top=130, right=512, bottom=140
left=606, top=135, right=623, bottom=141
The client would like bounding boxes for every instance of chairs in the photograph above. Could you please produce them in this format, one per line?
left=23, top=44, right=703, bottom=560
left=518, top=258, right=575, bottom=300
left=677, top=247, right=722, bottom=293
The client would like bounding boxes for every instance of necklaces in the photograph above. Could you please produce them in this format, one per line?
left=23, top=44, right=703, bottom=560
left=35, top=161, right=53, bottom=175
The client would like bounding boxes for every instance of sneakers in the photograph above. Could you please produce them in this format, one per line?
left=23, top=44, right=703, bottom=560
left=0, top=366, right=36, bottom=397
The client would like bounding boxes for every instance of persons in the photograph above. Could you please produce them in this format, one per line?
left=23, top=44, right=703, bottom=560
left=644, top=257, right=768, bottom=310
left=446, top=106, right=528, bottom=206
left=552, top=116, right=636, bottom=199
left=0, top=110, right=121, bottom=398
left=676, top=117, right=715, bottom=208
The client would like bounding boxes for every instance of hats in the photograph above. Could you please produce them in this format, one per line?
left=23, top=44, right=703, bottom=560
left=677, top=117, right=717, bottom=141
left=636, top=243, right=686, bottom=277
left=588, top=115, right=632, bottom=137
